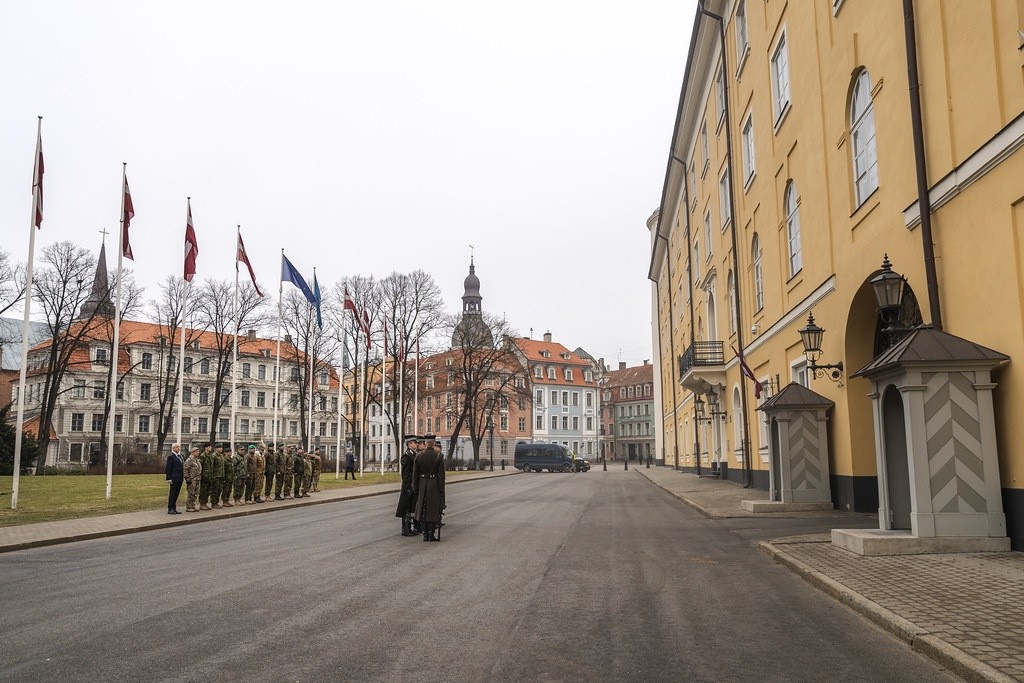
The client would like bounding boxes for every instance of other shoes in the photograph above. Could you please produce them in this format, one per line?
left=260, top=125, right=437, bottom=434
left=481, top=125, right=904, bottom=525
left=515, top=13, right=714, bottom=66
left=168, top=489, right=321, bottom=514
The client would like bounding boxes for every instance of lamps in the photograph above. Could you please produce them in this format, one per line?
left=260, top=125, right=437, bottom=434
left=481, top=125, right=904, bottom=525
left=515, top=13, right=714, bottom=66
left=871, top=252, right=934, bottom=345
left=706, top=385, right=728, bottom=424
left=693, top=393, right=712, bottom=428
left=798, top=311, right=843, bottom=387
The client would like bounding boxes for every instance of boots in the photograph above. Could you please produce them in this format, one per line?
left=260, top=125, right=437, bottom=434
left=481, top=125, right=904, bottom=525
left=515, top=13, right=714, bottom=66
left=423, top=532, right=427, bottom=541
left=412, top=519, right=423, bottom=533
left=428, top=532, right=436, bottom=541
left=401, top=519, right=417, bottom=536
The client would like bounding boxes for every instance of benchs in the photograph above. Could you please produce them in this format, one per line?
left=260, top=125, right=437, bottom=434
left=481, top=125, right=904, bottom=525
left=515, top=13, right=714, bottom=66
left=370, top=463, right=392, bottom=472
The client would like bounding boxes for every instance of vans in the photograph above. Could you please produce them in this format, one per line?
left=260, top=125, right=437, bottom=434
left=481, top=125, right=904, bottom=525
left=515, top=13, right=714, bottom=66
left=514, top=443, right=584, bottom=472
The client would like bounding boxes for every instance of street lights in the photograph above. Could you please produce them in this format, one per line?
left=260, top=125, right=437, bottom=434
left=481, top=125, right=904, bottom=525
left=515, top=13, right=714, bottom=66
left=490, top=430, right=493, bottom=470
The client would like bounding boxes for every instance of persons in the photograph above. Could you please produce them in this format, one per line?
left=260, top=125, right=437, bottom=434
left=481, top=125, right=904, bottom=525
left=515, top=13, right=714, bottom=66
left=166, top=443, right=186, bottom=514
left=211, top=443, right=234, bottom=509
left=253, top=443, right=284, bottom=503
left=199, top=442, right=213, bottom=510
left=284, top=445, right=322, bottom=499
left=344, top=448, right=357, bottom=480
left=233, top=445, right=261, bottom=505
left=183, top=447, right=202, bottom=512
left=394, top=434, right=445, bottom=541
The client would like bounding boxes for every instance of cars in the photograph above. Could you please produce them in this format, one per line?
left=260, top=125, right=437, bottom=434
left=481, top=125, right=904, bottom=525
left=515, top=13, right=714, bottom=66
left=582, top=461, right=590, bottom=472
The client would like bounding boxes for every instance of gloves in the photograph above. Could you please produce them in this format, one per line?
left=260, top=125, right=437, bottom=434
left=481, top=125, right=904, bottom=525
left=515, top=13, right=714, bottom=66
left=407, top=489, right=413, bottom=498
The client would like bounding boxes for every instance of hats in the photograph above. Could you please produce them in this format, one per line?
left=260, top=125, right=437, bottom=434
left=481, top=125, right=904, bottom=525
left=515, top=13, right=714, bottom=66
left=214, top=444, right=222, bottom=448
left=278, top=443, right=284, bottom=447
left=416, top=436, right=425, bottom=442
left=268, top=443, right=273, bottom=446
left=249, top=445, right=255, bottom=448
left=425, top=435, right=436, bottom=441
left=287, top=444, right=293, bottom=448
left=258, top=445, right=265, bottom=449
left=224, top=448, right=231, bottom=452
left=239, top=445, right=245, bottom=449
left=403, top=435, right=417, bottom=443
left=191, top=447, right=199, bottom=452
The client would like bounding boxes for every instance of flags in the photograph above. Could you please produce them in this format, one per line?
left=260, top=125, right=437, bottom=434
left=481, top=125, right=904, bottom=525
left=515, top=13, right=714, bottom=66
left=733, top=347, right=764, bottom=399
left=364, top=300, right=371, bottom=350
left=122, top=174, right=134, bottom=260
left=32, top=133, right=45, bottom=230
left=281, top=254, right=317, bottom=307
left=344, top=288, right=365, bottom=332
left=315, top=272, right=323, bottom=331
left=184, top=203, right=199, bottom=282
left=237, top=233, right=263, bottom=296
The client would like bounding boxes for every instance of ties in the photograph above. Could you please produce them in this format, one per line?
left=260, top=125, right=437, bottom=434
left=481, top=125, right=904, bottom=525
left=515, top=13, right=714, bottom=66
left=178, top=455, right=183, bottom=464
